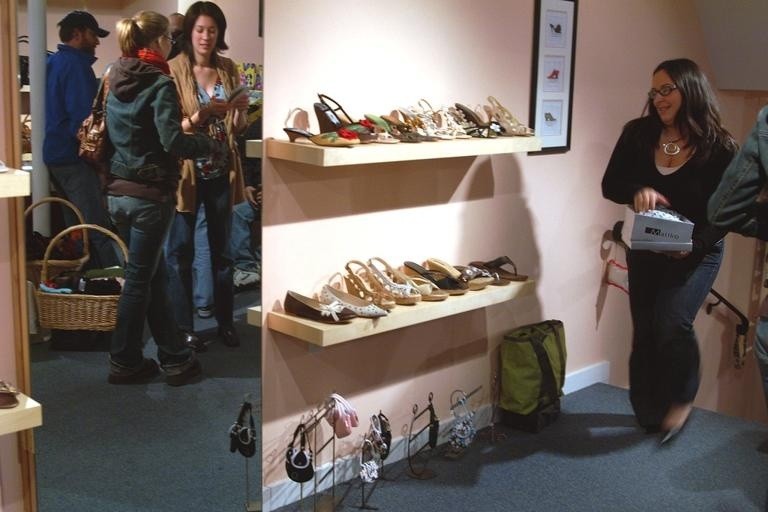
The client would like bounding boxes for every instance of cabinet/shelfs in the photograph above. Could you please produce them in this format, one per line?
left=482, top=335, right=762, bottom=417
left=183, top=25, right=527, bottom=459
left=263, top=130, right=543, bottom=350
left=0, top=164, right=47, bottom=454
left=242, top=134, right=266, bottom=332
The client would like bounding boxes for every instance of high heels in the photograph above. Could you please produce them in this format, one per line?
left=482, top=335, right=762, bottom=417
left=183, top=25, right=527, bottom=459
left=283, top=94, right=535, bottom=147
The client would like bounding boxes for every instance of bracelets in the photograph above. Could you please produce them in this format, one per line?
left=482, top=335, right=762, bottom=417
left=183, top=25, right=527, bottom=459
left=188, top=116, right=195, bottom=127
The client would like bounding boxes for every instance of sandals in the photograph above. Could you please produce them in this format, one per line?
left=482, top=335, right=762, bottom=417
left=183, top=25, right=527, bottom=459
left=284, top=256, right=527, bottom=323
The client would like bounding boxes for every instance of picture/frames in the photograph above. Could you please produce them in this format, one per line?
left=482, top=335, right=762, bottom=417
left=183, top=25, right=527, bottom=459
left=524, top=1, right=582, bottom=161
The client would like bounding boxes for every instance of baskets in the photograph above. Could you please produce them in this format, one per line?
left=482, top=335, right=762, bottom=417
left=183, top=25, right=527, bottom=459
left=34, top=224, right=129, bottom=332
left=24, top=197, right=90, bottom=290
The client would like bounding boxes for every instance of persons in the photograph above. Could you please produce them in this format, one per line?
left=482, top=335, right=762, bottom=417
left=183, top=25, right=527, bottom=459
left=42, top=12, right=118, bottom=353
left=706, top=107, right=768, bottom=456
left=101, top=10, right=203, bottom=387
left=166, top=1, right=251, bottom=353
left=227, top=117, right=262, bottom=295
left=166, top=13, right=185, bottom=55
left=600, top=55, right=741, bottom=451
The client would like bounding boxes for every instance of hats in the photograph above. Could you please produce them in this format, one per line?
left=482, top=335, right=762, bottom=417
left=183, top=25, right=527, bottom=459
left=57, top=10, right=110, bottom=38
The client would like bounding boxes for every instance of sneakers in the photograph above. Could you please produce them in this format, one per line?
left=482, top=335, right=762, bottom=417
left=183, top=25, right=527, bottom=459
left=232, top=267, right=261, bottom=288
left=197, top=304, right=214, bottom=319
left=165, top=359, right=200, bottom=385
left=108, top=355, right=158, bottom=384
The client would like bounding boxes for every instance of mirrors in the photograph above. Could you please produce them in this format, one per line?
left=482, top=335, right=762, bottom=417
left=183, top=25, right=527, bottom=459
left=6, top=2, right=267, bottom=512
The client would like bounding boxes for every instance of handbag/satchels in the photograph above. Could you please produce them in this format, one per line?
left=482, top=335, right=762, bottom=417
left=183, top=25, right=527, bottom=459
left=497, top=319, right=566, bottom=434
left=77, top=109, right=114, bottom=167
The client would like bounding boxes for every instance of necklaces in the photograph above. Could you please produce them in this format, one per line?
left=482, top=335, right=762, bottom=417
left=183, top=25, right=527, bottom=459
left=661, top=126, right=688, bottom=157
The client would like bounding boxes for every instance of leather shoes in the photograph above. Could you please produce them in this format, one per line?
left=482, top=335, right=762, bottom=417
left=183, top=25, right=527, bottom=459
left=182, top=331, right=204, bottom=354
left=217, top=324, right=240, bottom=347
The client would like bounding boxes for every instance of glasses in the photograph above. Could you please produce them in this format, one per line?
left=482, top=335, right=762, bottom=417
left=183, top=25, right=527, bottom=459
left=648, top=86, right=677, bottom=101
left=160, top=32, right=177, bottom=45
